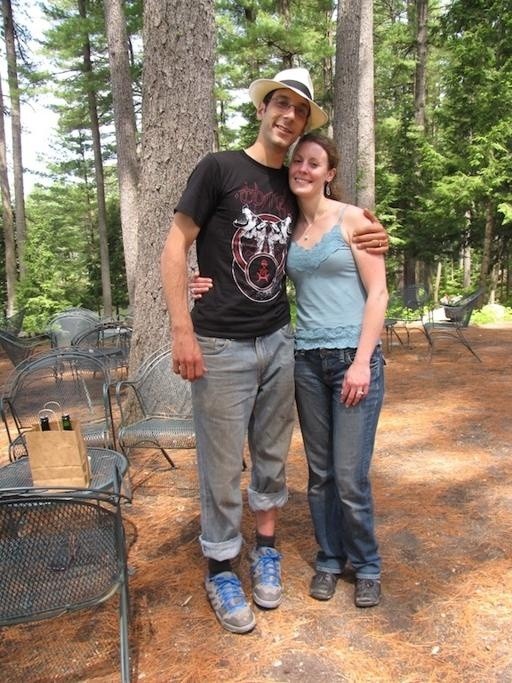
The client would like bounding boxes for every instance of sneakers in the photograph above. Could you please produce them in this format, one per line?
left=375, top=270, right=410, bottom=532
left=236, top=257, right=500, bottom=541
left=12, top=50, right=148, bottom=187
left=354, top=578, right=381, bottom=607
left=249, top=542, right=283, bottom=609
left=204, top=567, right=256, bottom=633
left=310, top=571, right=341, bottom=600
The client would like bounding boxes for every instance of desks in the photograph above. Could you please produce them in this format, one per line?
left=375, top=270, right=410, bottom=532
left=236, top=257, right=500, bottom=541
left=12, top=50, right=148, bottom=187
left=99, top=327, right=130, bottom=340
left=383, top=319, right=398, bottom=354
left=0, top=446, right=129, bottom=529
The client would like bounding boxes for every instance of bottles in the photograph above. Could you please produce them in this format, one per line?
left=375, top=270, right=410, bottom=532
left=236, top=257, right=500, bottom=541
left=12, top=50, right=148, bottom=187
left=40, top=416, right=51, bottom=430
left=62, top=414, right=73, bottom=431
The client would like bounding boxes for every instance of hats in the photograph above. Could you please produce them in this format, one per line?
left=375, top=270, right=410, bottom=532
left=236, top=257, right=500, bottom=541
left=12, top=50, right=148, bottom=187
left=249, top=67, right=327, bottom=138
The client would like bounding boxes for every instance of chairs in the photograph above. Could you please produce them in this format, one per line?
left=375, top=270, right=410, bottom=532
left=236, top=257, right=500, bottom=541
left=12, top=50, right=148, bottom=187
left=0, top=484, right=138, bottom=683
left=0, top=307, right=28, bottom=356
left=114, top=341, right=248, bottom=500
left=423, top=287, right=487, bottom=366
left=44, top=307, right=103, bottom=400
left=0, top=329, right=63, bottom=413
left=386, top=283, right=435, bottom=350
left=72, top=322, right=133, bottom=417
left=0, top=345, right=134, bottom=505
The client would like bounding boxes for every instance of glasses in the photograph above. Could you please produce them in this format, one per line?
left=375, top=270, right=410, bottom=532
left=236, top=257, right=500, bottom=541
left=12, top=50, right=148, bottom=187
left=271, top=94, right=308, bottom=118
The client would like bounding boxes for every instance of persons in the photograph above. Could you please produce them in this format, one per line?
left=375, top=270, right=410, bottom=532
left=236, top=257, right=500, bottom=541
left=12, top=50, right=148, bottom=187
left=157, top=66, right=390, bottom=635
left=187, top=131, right=391, bottom=608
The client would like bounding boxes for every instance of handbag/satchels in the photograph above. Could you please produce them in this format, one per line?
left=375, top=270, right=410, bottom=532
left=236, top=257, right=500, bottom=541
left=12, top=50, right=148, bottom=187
left=25, top=400, right=91, bottom=492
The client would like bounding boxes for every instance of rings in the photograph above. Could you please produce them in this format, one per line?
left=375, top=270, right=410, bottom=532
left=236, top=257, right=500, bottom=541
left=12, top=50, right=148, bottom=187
left=359, top=391, right=365, bottom=395
left=379, top=240, right=382, bottom=247
left=192, top=288, right=195, bottom=294
